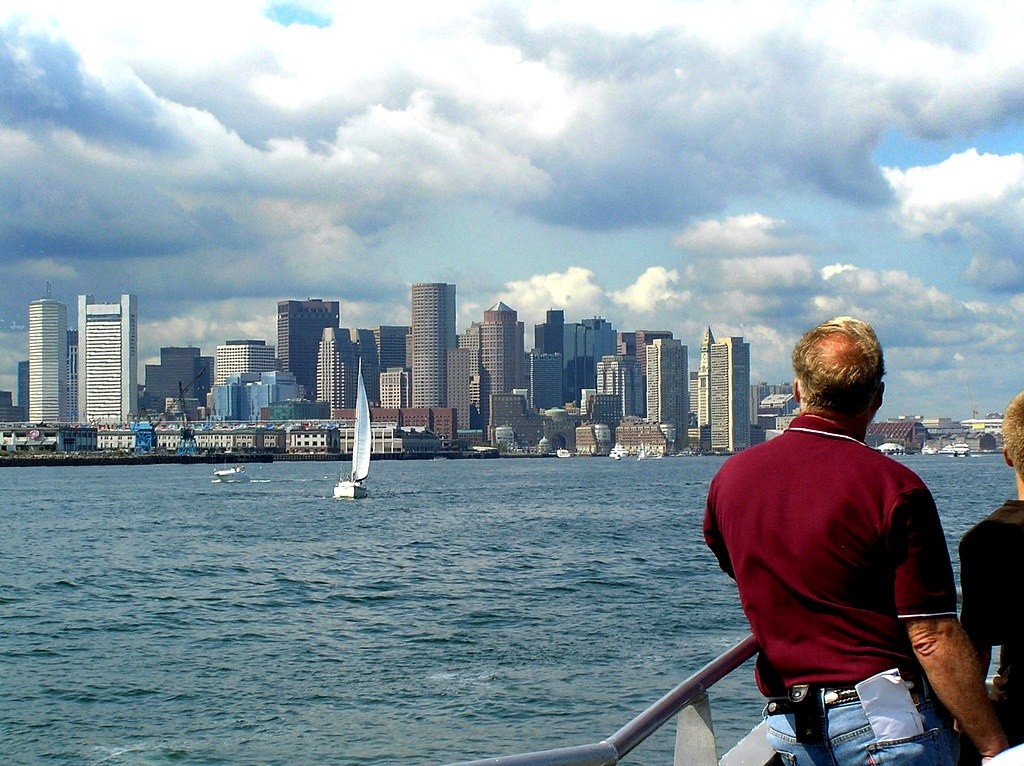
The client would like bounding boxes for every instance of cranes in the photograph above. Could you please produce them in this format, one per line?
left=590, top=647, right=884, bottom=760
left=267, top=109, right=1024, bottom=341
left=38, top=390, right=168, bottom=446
left=136, top=367, right=207, bottom=439
left=966, top=382, right=979, bottom=420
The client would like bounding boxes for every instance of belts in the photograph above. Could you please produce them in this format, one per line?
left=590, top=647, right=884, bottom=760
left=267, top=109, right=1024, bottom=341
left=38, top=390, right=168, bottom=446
left=766, top=679, right=924, bottom=717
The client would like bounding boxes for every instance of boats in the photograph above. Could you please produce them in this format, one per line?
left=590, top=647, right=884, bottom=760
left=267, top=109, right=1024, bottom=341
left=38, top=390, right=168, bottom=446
left=921, top=443, right=970, bottom=457
left=615, top=455, right=621, bottom=460
left=215, top=465, right=251, bottom=483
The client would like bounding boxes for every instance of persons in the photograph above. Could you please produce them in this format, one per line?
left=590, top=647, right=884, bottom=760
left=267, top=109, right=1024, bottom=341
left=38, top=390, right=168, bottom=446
left=955, top=390, right=1024, bottom=766
left=702, top=317, right=1008, bottom=766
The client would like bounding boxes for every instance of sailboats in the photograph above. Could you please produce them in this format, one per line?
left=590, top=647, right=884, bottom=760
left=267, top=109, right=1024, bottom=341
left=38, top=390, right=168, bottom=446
left=334, top=355, right=371, bottom=499
